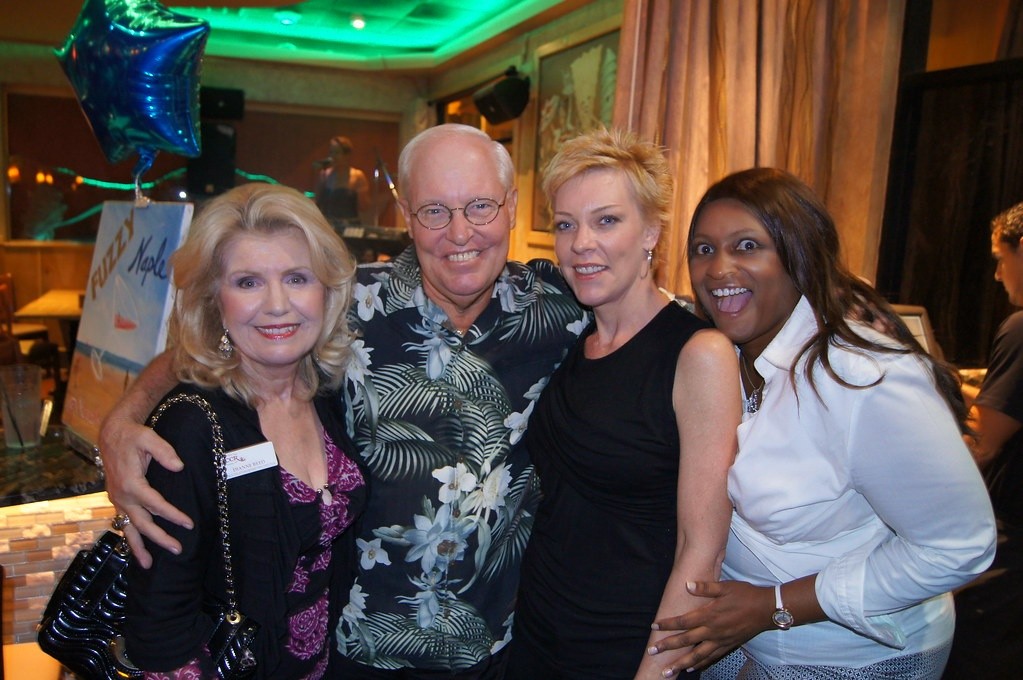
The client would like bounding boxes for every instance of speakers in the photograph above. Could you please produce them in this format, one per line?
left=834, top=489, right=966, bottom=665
left=186, top=84, right=245, bottom=197
left=472, top=78, right=530, bottom=126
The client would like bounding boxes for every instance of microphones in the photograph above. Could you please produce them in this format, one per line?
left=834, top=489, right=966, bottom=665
left=311, top=157, right=335, bottom=169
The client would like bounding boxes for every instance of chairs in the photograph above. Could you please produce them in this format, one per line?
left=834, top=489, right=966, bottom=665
left=1, top=270, right=51, bottom=352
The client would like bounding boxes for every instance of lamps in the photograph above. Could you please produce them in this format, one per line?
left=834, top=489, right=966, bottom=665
left=352, top=18, right=365, bottom=29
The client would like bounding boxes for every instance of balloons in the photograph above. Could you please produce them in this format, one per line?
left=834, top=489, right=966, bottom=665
left=52, top=0, right=210, bottom=184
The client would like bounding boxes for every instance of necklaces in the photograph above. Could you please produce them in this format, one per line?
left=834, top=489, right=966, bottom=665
left=740, top=353, right=765, bottom=412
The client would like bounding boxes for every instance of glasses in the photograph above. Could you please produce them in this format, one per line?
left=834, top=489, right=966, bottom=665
left=409, top=185, right=511, bottom=231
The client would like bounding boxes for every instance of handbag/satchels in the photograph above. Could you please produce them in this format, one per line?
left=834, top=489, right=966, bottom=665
left=35, top=393, right=256, bottom=678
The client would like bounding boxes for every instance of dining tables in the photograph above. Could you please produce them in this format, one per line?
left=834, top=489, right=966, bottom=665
left=16, top=285, right=88, bottom=377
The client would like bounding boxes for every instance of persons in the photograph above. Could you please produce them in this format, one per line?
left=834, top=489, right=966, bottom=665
left=960, top=201, right=1022, bottom=528
left=316, top=135, right=368, bottom=226
left=645, top=167, right=996, bottom=680
left=95, top=125, right=598, bottom=680
left=128, top=182, right=373, bottom=680
left=511, top=130, right=743, bottom=680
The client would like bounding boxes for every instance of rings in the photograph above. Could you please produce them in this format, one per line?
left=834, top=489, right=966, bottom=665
left=111, top=515, right=132, bottom=532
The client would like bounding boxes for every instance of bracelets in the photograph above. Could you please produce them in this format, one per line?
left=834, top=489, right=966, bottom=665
left=771, top=584, right=794, bottom=630
